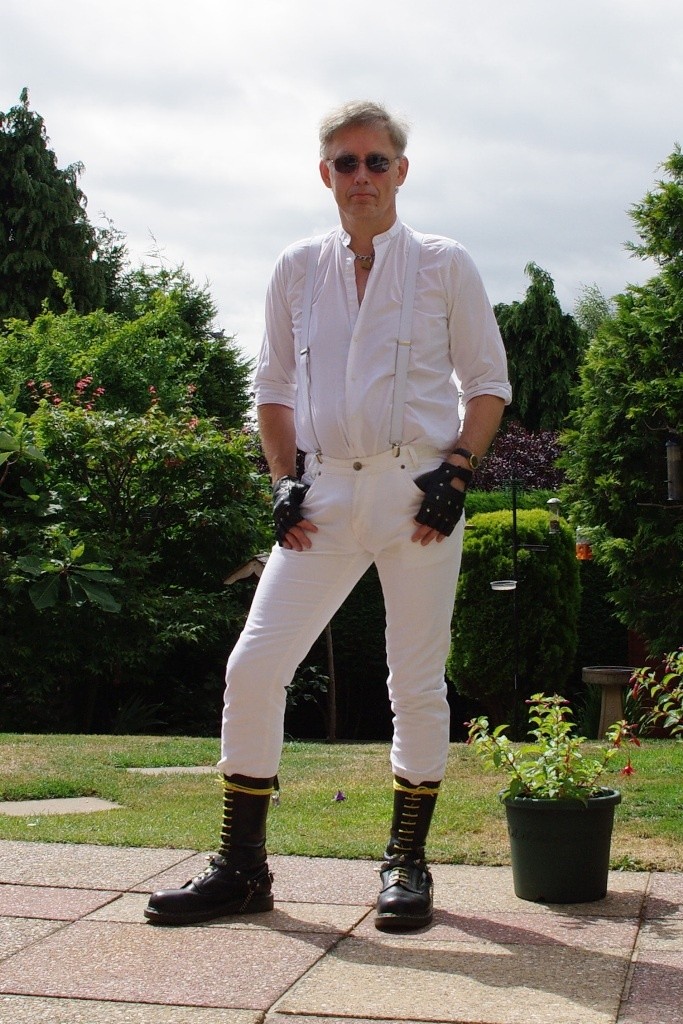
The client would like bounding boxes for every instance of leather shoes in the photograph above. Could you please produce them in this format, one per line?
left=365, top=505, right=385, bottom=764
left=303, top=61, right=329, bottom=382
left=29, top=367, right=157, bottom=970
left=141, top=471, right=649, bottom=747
left=144, top=851, right=274, bottom=923
left=374, top=858, right=433, bottom=931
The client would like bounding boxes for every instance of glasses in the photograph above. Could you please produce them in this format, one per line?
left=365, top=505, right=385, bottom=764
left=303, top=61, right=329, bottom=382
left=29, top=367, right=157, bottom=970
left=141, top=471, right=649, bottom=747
left=328, top=154, right=400, bottom=174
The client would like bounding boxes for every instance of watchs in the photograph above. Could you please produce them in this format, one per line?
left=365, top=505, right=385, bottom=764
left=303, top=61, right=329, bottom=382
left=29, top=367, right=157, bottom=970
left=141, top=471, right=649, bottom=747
left=451, top=447, right=479, bottom=469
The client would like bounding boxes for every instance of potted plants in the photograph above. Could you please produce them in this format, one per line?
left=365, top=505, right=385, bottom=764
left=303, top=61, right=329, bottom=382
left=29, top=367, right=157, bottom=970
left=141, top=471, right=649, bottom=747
left=463, top=692, right=641, bottom=903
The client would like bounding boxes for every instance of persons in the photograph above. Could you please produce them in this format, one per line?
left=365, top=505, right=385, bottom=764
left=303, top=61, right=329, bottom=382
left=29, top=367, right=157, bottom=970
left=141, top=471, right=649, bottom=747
left=143, top=101, right=513, bottom=930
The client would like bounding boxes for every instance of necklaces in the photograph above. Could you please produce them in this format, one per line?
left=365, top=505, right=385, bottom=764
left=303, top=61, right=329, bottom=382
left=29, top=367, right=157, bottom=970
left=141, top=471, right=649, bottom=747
left=356, top=252, right=375, bottom=271
